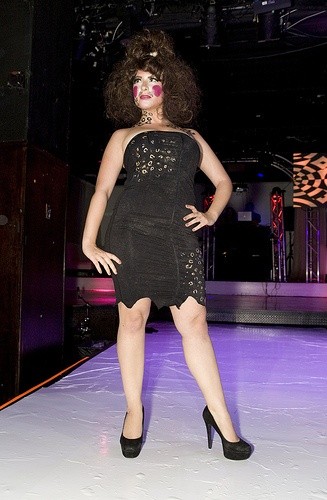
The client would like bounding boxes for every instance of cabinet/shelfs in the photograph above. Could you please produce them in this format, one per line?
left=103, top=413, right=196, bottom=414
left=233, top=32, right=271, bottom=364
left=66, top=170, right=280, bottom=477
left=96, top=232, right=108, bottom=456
left=1, top=139, right=69, bottom=394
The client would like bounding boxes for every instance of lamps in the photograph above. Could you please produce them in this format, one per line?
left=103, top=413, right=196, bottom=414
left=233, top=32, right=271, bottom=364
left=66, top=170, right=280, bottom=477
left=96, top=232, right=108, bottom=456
left=232, top=184, right=248, bottom=193
left=140, top=0, right=282, bottom=49
left=72, top=11, right=113, bottom=94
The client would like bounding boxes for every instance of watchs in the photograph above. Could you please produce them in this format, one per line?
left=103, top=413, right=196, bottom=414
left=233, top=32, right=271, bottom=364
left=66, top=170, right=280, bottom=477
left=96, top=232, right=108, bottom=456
left=82, top=30, right=251, bottom=460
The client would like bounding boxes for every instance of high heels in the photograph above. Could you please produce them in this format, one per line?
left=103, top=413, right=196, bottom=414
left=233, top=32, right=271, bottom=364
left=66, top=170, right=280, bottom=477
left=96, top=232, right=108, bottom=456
left=201, top=404, right=252, bottom=460
left=120, top=405, right=144, bottom=458
